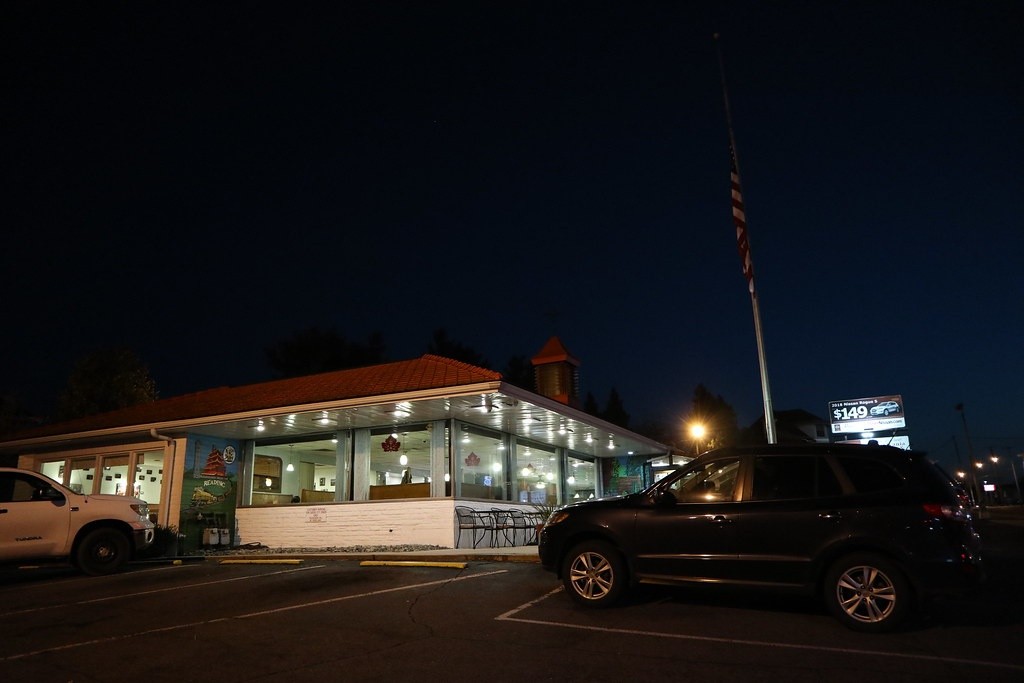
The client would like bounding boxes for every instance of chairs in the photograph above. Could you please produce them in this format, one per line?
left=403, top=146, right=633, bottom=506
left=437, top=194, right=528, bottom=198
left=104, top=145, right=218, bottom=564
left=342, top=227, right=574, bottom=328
left=454, top=505, right=493, bottom=550
left=491, top=508, right=516, bottom=546
left=509, top=509, right=528, bottom=546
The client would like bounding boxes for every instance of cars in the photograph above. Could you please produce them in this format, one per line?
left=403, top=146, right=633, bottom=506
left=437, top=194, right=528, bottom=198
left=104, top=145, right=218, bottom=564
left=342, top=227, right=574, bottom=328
left=537, top=442, right=984, bottom=633
left=0, top=466, right=158, bottom=576
left=870, top=401, right=901, bottom=417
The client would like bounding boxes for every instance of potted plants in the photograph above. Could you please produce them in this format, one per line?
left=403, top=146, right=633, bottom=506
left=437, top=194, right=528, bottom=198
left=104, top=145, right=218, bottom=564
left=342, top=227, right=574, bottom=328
left=532, top=497, right=555, bottom=544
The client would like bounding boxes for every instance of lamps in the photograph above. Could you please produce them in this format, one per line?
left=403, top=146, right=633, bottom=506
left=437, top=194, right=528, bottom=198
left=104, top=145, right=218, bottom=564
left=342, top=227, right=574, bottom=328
left=536, top=474, right=545, bottom=489
left=265, top=460, right=273, bottom=487
left=492, top=442, right=502, bottom=472
left=287, top=445, right=295, bottom=471
left=521, top=455, right=529, bottom=476
left=568, top=460, right=575, bottom=484
left=546, top=456, right=554, bottom=480
left=400, top=433, right=408, bottom=465
